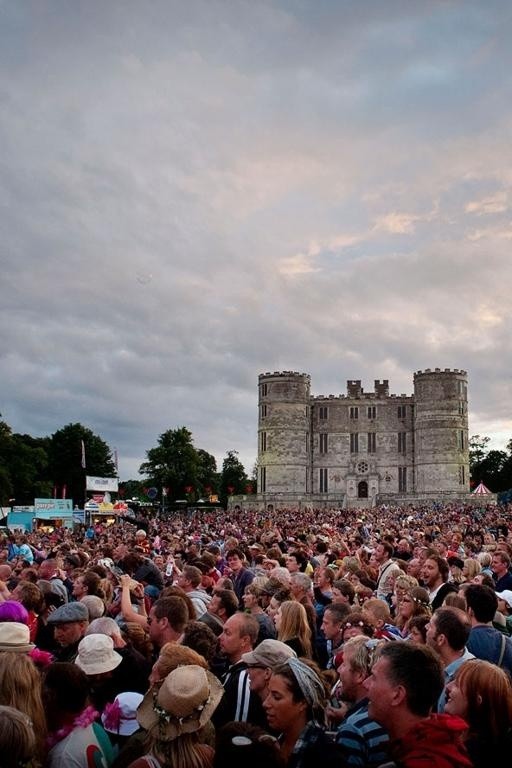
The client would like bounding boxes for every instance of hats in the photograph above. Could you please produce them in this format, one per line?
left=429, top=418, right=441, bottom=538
left=495, top=587, right=512, bottom=610
left=100, top=691, right=148, bottom=739
left=1, top=620, right=39, bottom=656
left=242, top=638, right=300, bottom=672
left=47, top=600, right=91, bottom=628
left=135, top=659, right=226, bottom=740
left=74, top=633, right=122, bottom=677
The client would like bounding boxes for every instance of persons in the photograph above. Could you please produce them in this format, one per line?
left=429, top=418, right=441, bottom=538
left=101, top=692, right=145, bottom=736
left=407, top=558, right=420, bottom=575
left=217, top=612, right=259, bottom=722
left=463, top=585, right=512, bottom=675
left=146, top=597, right=209, bottom=660
left=241, top=638, right=297, bottom=702
left=362, top=600, right=404, bottom=641
left=494, top=590, right=512, bottom=633
left=120, top=582, right=196, bottom=638
left=243, top=585, right=268, bottom=623
left=361, top=642, right=472, bottom=768
left=462, top=559, right=481, bottom=584
left=1, top=601, right=29, bottom=625
left=425, top=607, right=478, bottom=715
left=320, top=670, right=338, bottom=689
left=214, top=577, right=231, bottom=592
left=306, top=508, right=374, bottom=556
left=410, top=499, right=511, bottom=553
left=199, top=551, right=221, bottom=584
left=178, top=621, right=218, bottom=664
left=395, top=587, right=431, bottom=636
left=394, top=575, right=418, bottom=601
left=149, top=642, right=207, bottom=687
left=189, top=551, right=200, bottom=563
left=395, top=539, right=410, bottom=559
left=340, top=613, right=374, bottom=645
left=207, top=587, right=240, bottom=621
left=258, top=544, right=289, bottom=588
left=477, top=554, right=493, bottom=573
left=41, top=662, right=115, bottom=767
left=127, top=665, right=224, bottom=768
left=423, top=556, right=458, bottom=605
left=1, top=706, right=40, bottom=768
left=321, top=603, right=351, bottom=649
left=408, top=615, right=431, bottom=645
left=471, top=573, right=494, bottom=587
left=0, top=652, right=48, bottom=762
left=188, top=507, right=306, bottom=548
left=212, top=721, right=282, bottom=768
left=226, top=550, right=256, bottom=590
left=267, top=590, right=288, bottom=618
left=288, top=573, right=316, bottom=638
left=262, top=657, right=335, bottom=768
left=492, top=551, right=512, bottom=591
left=1, top=520, right=119, bottom=606
left=375, top=542, right=400, bottom=600
left=317, top=556, right=378, bottom=604
left=274, top=601, right=312, bottom=660
left=442, top=592, right=467, bottom=612
left=494, top=610, right=506, bottom=631
left=179, top=566, right=211, bottom=620
left=118, top=499, right=188, bottom=581
left=443, top=659, right=512, bottom=768
left=74, top=634, right=124, bottom=685
left=84, top=618, right=146, bottom=674
left=81, top=596, right=104, bottom=623
left=286, top=551, right=307, bottom=574
left=0, top=621, right=36, bottom=655
left=376, top=500, right=410, bottom=541
left=46, top=602, right=89, bottom=664
left=335, top=635, right=391, bottom=768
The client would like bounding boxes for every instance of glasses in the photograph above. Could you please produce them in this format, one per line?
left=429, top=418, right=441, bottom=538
left=244, top=663, right=272, bottom=670
